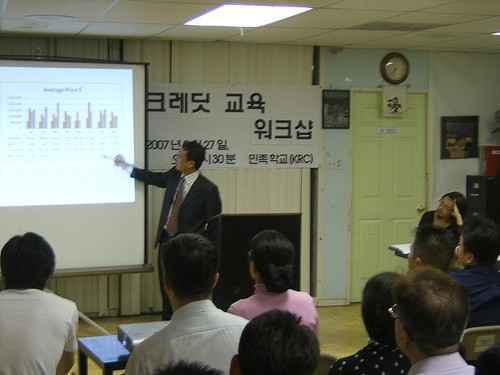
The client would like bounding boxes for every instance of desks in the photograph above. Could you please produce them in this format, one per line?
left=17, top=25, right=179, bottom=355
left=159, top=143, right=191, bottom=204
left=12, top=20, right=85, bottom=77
left=77, top=335, right=131, bottom=375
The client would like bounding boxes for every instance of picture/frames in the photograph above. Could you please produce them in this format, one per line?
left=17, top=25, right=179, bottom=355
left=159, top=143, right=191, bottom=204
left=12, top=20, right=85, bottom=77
left=322, top=89, right=351, bottom=130
left=440, top=116, right=480, bottom=159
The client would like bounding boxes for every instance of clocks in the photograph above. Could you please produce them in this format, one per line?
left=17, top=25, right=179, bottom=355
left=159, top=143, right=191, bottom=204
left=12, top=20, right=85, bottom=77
left=379, top=52, right=410, bottom=85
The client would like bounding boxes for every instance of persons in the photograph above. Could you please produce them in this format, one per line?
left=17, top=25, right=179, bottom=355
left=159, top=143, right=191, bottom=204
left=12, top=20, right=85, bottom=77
left=123, top=232, right=250, bottom=375
left=229, top=309, right=320, bottom=375
left=114, top=141, right=223, bottom=320
left=0, top=232, right=79, bottom=375
left=408, top=190, right=500, bottom=327
left=227, top=231, right=319, bottom=341
left=327, top=267, right=500, bottom=375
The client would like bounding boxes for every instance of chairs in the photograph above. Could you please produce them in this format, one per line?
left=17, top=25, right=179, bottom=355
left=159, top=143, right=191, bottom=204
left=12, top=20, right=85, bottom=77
left=458, top=326, right=500, bottom=363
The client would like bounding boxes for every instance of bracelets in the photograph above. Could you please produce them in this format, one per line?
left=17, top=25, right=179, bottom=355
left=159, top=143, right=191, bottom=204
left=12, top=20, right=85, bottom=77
left=455, top=246, right=460, bottom=257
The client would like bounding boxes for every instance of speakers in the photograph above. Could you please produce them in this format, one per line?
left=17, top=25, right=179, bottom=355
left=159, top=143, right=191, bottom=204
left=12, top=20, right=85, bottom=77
left=466, top=174, right=500, bottom=222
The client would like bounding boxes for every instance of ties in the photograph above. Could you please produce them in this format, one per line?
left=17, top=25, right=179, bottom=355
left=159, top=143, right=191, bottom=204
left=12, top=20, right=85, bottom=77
left=166, top=177, right=186, bottom=236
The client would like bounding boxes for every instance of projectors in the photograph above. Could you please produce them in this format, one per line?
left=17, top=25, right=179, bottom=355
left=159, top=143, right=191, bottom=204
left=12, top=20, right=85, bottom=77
left=117, top=320, right=171, bottom=354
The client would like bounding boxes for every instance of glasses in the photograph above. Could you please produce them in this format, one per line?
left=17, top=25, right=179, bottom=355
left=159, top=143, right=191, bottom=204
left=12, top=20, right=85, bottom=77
left=247, top=249, right=253, bottom=260
left=439, top=199, right=452, bottom=211
left=388, top=304, right=414, bottom=341
